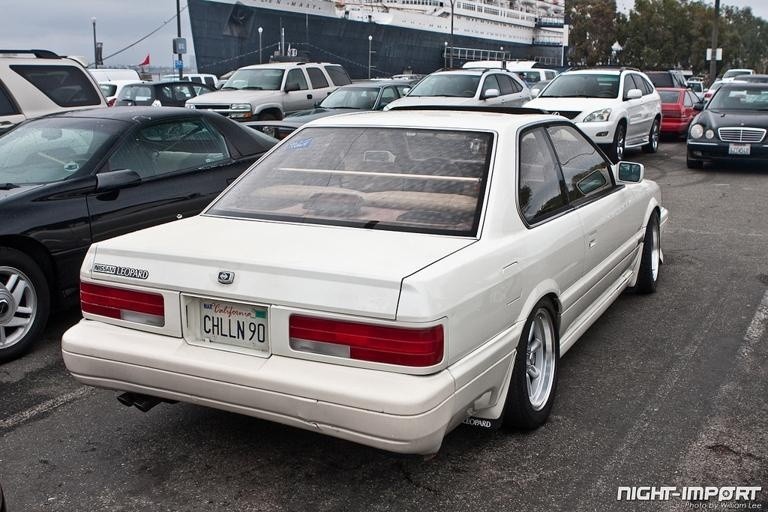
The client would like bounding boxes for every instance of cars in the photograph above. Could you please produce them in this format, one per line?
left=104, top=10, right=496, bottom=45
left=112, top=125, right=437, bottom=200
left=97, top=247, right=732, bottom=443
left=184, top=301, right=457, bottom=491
left=61, top=109, right=669, bottom=455
left=0, top=106, right=280, bottom=366
left=644, top=69, right=768, bottom=167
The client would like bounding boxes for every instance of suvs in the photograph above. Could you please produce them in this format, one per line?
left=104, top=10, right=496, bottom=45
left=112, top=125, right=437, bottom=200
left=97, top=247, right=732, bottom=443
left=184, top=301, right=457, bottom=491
left=0, top=49, right=110, bottom=136
left=89, top=63, right=664, bottom=162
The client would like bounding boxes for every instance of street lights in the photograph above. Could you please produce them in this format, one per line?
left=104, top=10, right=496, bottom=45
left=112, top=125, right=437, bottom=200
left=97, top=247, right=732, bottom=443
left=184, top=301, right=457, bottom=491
left=368, top=34, right=373, bottom=78
left=444, top=41, right=449, bottom=68
left=258, top=26, right=263, bottom=63
left=500, top=46, right=504, bottom=58
left=90, top=17, right=98, bottom=70
